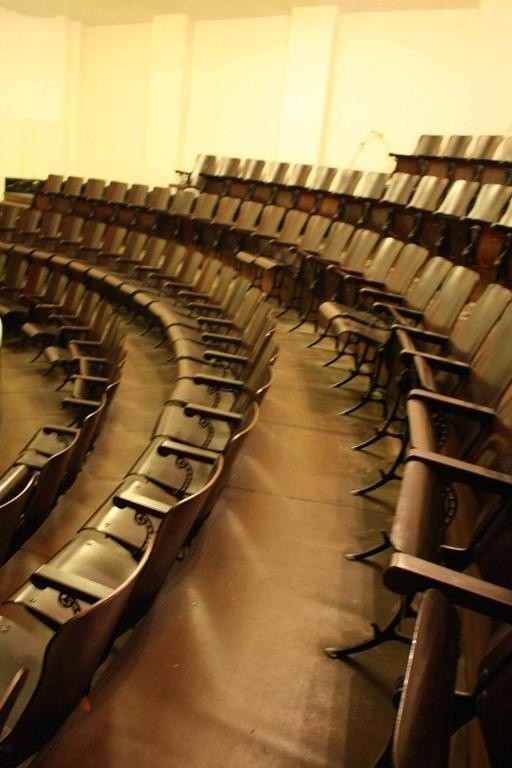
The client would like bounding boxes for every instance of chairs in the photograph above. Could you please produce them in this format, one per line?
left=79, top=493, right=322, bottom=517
left=0, top=134, right=511, bottom=766
